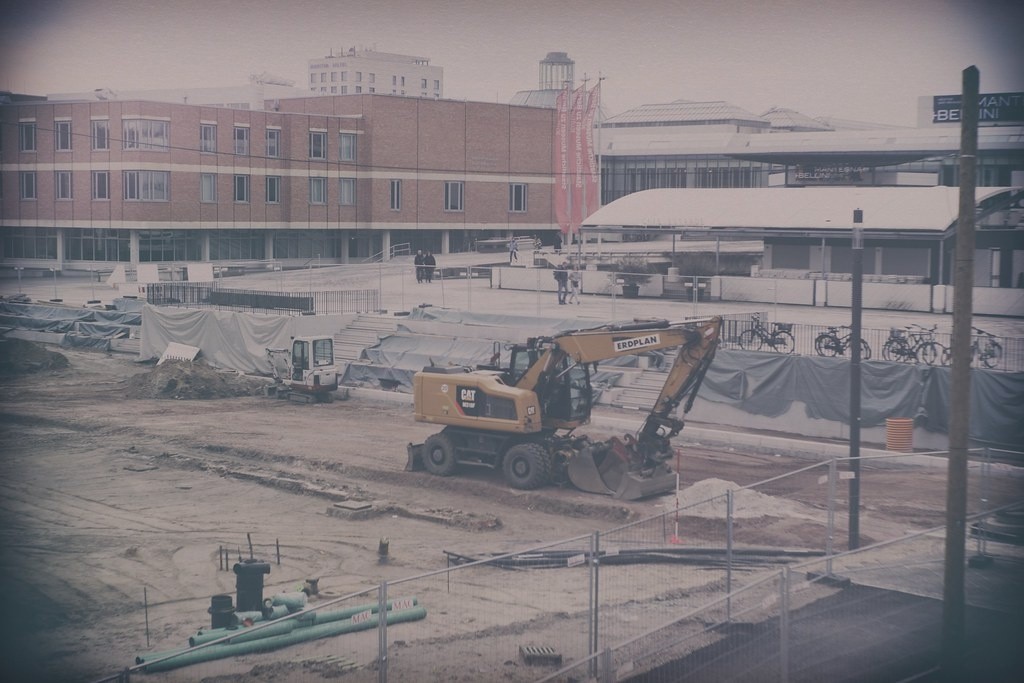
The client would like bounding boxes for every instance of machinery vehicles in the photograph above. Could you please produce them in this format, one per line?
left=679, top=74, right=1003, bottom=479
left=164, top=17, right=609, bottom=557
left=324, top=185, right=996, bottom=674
left=400, top=313, right=726, bottom=502
left=266, top=336, right=342, bottom=404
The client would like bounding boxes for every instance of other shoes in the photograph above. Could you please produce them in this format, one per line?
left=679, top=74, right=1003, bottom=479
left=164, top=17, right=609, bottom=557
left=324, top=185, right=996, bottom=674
left=569, top=301, right=573, bottom=305
left=429, top=281, right=432, bottom=283
left=426, top=281, right=428, bottom=283
left=417, top=281, right=420, bottom=284
left=421, top=281, right=423, bottom=283
left=577, top=300, right=580, bottom=305
left=559, top=300, right=566, bottom=305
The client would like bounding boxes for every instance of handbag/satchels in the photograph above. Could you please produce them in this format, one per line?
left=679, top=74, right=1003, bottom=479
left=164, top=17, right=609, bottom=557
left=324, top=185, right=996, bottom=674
left=573, top=280, right=578, bottom=287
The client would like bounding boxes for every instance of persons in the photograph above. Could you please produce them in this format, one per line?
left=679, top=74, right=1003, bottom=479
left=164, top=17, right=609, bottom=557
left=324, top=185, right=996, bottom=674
left=533, top=231, right=563, bottom=253
left=508, top=238, right=518, bottom=263
left=553, top=262, right=569, bottom=305
left=568, top=268, right=581, bottom=306
left=413, top=249, right=425, bottom=283
left=423, top=251, right=436, bottom=283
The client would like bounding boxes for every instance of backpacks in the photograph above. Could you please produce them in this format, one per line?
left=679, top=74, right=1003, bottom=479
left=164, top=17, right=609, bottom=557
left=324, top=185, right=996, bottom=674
left=553, top=264, right=562, bottom=280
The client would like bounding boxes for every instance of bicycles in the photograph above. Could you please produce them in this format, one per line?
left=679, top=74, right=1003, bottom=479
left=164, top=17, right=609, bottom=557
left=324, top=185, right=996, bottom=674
left=738, top=311, right=798, bottom=354
left=971, top=326, right=1003, bottom=369
left=815, top=323, right=952, bottom=367
